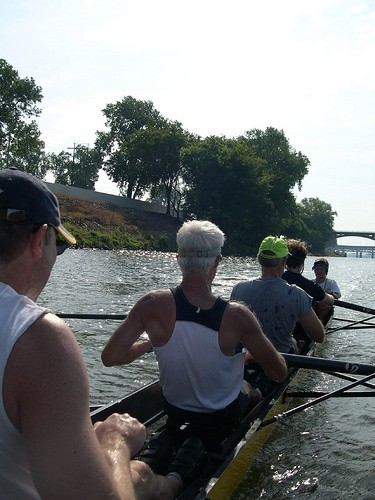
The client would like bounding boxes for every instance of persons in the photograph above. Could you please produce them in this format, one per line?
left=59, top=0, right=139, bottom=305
left=102, top=221, right=286, bottom=445
left=0, top=167, right=201, bottom=500
left=229, top=235, right=341, bottom=398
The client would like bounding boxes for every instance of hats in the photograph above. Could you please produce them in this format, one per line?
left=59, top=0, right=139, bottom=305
left=258, top=236, right=292, bottom=259
left=0, top=167, right=77, bottom=245
left=312, top=264, right=328, bottom=270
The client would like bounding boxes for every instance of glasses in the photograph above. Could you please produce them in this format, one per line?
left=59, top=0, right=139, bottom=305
left=33, top=223, right=68, bottom=256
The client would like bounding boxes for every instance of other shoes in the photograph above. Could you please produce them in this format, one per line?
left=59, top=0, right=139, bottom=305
left=139, top=433, right=210, bottom=500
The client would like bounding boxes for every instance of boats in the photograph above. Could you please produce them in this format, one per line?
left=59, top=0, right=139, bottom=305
left=84, top=306, right=335, bottom=500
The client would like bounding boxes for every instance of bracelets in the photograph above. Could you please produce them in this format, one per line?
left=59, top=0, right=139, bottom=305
left=143, top=338, right=155, bottom=354
